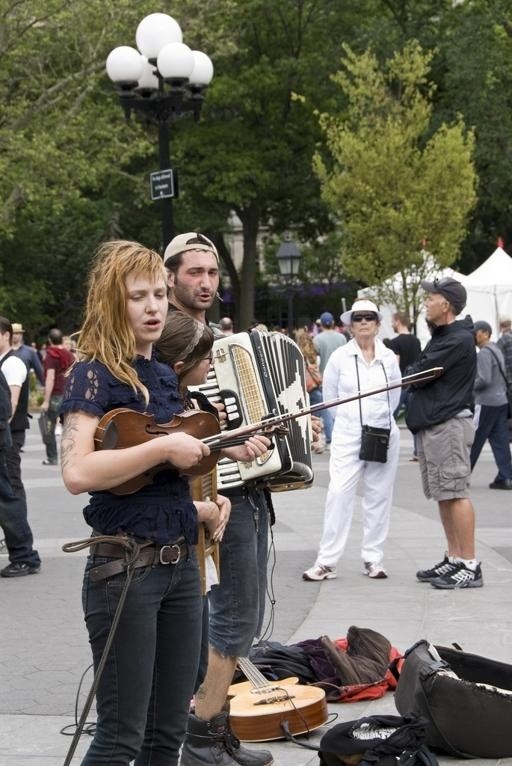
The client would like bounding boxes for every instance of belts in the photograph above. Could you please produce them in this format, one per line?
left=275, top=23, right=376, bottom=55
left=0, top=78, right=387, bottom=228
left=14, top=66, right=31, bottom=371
left=88, top=541, right=188, bottom=583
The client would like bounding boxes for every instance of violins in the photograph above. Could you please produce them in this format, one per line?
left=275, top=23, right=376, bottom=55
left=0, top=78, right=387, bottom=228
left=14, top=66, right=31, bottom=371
left=95, top=407, right=290, bottom=495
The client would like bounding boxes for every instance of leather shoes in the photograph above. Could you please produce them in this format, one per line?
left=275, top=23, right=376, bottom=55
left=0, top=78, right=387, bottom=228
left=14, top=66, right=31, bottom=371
left=489, top=471, right=511, bottom=490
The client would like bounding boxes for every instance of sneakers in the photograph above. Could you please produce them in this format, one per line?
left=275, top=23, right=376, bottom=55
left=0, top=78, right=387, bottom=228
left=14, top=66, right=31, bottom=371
left=431, top=561, right=483, bottom=589
left=303, top=563, right=337, bottom=580
left=417, top=551, right=454, bottom=582
left=363, top=560, right=388, bottom=578
left=1, top=560, right=39, bottom=576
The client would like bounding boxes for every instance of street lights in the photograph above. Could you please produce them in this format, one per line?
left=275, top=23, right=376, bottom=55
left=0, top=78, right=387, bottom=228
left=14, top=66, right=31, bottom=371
left=105, top=9, right=217, bottom=252
left=276, top=228, right=302, bottom=340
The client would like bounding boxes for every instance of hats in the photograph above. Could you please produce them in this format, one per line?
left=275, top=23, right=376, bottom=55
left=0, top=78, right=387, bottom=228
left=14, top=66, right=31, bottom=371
left=422, top=277, right=466, bottom=309
left=164, top=232, right=219, bottom=264
left=321, top=312, right=334, bottom=322
left=340, top=299, right=383, bottom=324
left=11, top=323, right=26, bottom=333
left=474, top=321, right=492, bottom=332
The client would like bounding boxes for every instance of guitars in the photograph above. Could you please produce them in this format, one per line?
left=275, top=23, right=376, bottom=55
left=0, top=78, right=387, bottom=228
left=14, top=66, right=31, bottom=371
left=227, top=657, right=328, bottom=741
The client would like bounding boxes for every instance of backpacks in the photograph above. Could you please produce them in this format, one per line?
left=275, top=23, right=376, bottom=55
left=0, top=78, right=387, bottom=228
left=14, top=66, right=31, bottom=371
left=390, top=640, right=511, bottom=759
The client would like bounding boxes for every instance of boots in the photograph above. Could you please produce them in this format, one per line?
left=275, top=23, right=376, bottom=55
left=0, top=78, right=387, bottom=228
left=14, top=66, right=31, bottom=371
left=180, top=701, right=274, bottom=765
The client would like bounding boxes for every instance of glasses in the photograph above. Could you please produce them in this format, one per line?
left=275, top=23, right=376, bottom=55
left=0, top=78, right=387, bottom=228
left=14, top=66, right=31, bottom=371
left=351, top=314, right=377, bottom=321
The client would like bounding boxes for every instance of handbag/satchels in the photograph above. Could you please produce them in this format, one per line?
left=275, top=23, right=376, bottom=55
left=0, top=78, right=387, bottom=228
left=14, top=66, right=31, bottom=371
left=38, top=415, right=53, bottom=444
left=359, top=425, right=390, bottom=463
left=506, top=380, right=512, bottom=418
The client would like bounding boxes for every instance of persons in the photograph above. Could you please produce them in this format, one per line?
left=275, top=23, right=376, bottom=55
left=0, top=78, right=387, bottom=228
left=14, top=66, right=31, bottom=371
left=302, top=300, right=402, bottom=580
left=51, top=236, right=274, bottom=766
left=125, top=310, right=232, bottom=725
left=0, top=316, right=41, bottom=576
left=160, top=230, right=324, bottom=764
left=274, top=313, right=512, bottom=490
left=11, top=323, right=76, bottom=464
left=404, top=280, right=485, bottom=588
